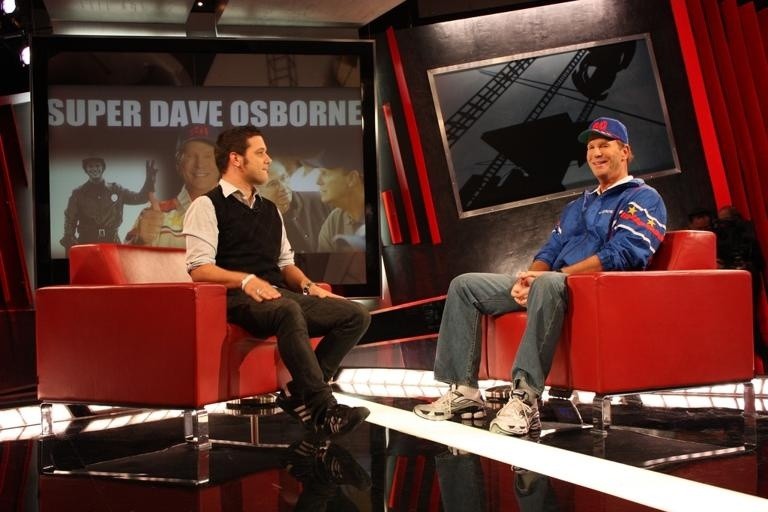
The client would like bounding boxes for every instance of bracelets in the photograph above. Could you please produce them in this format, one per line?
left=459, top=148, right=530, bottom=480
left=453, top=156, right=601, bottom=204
left=555, top=269, right=563, bottom=273
left=240, top=274, right=257, bottom=291
left=301, top=282, right=315, bottom=296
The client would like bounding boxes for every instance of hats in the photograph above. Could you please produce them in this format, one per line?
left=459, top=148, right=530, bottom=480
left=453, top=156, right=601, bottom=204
left=177, top=123, right=218, bottom=150
left=303, top=145, right=363, bottom=169
left=578, top=117, right=628, bottom=144
left=82, top=155, right=105, bottom=172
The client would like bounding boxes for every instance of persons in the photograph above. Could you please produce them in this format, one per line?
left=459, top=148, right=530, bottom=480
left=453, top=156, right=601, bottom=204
left=179, top=125, right=371, bottom=442
left=412, top=116, right=669, bottom=435
left=285, top=158, right=322, bottom=188
left=716, top=203, right=768, bottom=377
left=124, top=124, right=220, bottom=246
left=434, top=447, right=559, bottom=512
left=686, top=207, right=712, bottom=232
left=312, top=165, right=367, bottom=253
left=271, top=431, right=373, bottom=511
left=258, top=160, right=333, bottom=254
left=59, top=157, right=160, bottom=256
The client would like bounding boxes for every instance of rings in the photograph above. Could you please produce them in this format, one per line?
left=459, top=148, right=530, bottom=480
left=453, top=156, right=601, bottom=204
left=256, top=289, right=263, bottom=295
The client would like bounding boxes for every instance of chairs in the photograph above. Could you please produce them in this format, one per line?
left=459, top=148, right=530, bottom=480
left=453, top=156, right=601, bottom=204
left=450, top=227, right=756, bottom=427
left=32, top=242, right=333, bottom=447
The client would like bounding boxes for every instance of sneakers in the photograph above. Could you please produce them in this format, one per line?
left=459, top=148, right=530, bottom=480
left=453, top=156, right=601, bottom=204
left=490, top=390, right=541, bottom=435
left=312, top=404, right=370, bottom=442
left=276, top=381, right=312, bottom=432
left=315, top=438, right=372, bottom=493
left=414, top=384, right=487, bottom=420
left=276, top=435, right=318, bottom=470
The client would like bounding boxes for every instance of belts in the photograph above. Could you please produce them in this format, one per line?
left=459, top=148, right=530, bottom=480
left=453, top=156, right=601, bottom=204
left=82, top=227, right=117, bottom=237
